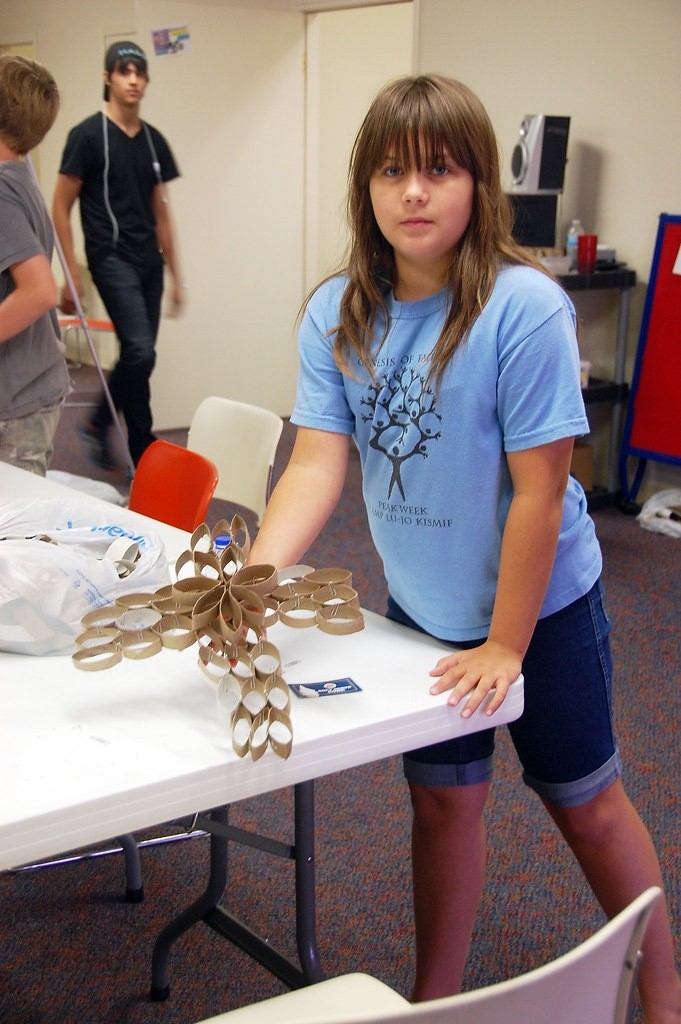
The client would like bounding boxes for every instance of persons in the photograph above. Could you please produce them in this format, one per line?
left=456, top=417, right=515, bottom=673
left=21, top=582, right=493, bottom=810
left=0, top=56, right=73, bottom=477
left=51, top=41, right=186, bottom=480
left=202, top=73, right=681, bottom=1024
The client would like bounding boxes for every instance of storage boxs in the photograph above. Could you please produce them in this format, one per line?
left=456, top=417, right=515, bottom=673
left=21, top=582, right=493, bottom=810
left=569, top=443, right=594, bottom=493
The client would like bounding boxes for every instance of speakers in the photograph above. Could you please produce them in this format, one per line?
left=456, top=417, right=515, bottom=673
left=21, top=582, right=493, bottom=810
left=503, top=193, right=558, bottom=249
left=511, top=113, right=571, bottom=194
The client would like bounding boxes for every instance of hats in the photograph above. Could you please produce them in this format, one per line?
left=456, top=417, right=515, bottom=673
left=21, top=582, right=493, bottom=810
left=103, top=40, right=147, bottom=101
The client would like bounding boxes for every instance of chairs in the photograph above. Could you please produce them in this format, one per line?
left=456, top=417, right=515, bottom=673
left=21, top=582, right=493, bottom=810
left=125, top=439, right=217, bottom=533
left=193, top=886, right=662, bottom=1024
left=55, top=315, right=118, bottom=408
left=188, top=395, right=285, bottom=527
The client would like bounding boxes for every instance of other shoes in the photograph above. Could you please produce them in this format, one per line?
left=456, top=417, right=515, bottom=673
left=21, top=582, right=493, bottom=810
left=76, top=420, right=117, bottom=469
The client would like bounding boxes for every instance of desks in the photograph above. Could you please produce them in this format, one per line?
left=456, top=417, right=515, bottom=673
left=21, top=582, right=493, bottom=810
left=0, top=456, right=524, bottom=997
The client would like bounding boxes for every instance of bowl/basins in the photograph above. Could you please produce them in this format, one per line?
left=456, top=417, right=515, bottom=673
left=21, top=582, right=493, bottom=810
left=536, top=256, right=573, bottom=275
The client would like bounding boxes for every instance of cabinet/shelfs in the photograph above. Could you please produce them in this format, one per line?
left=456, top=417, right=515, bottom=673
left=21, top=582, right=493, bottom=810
left=553, top=266, right=636, bottom=513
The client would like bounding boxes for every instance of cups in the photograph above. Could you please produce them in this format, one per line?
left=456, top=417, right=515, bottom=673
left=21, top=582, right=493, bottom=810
left=577, top=234, right=598, bottom=274
left=567, top=219, right=586, bottom=271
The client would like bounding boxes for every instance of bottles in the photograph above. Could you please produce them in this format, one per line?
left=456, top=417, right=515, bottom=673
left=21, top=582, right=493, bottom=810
left=204, top=536, right=238, bottom=579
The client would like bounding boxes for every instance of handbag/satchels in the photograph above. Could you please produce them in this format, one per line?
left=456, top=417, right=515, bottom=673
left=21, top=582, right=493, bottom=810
left=637, top=486, right=681, bottom=542
left=0, top=488, right=180, bottom=656
